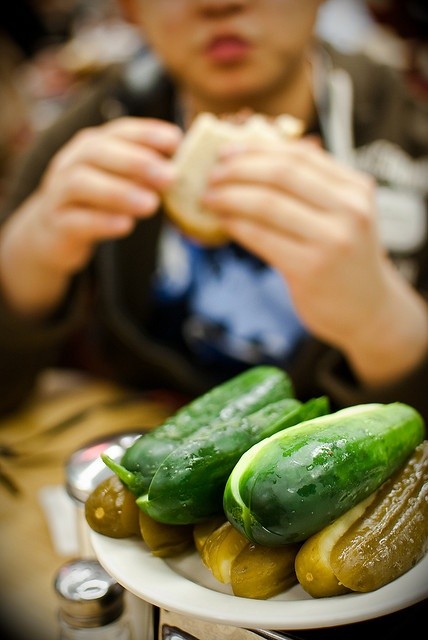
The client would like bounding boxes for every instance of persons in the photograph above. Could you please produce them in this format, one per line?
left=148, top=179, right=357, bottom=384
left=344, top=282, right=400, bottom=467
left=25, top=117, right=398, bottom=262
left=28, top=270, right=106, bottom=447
left=2, top=0, right=427, bottom=418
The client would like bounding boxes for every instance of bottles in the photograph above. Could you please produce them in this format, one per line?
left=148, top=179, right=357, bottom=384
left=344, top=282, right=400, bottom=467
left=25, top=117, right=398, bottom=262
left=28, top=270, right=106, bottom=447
left=51, top=556, right=134, bottom=639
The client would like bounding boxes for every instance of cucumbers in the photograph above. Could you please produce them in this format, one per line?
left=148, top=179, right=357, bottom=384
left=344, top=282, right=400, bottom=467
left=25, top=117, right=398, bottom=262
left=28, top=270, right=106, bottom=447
left=100, top=367, right=329, bottom=525
left=295, top=440, right=428, bottom=599
left=87, top=474, right=193, bottom=557
left=224, top=402, right=425, bottom=546
left=193, top=519, right=300, bottom=598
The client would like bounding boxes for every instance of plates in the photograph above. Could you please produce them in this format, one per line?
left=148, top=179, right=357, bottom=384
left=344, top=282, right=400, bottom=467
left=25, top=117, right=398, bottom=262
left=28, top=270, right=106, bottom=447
left=84, top=436, right=427, bottom=631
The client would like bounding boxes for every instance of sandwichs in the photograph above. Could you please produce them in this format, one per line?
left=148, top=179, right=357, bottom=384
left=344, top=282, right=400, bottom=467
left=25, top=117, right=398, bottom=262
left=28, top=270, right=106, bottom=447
left=162, top=108, right=306, bottom=248
